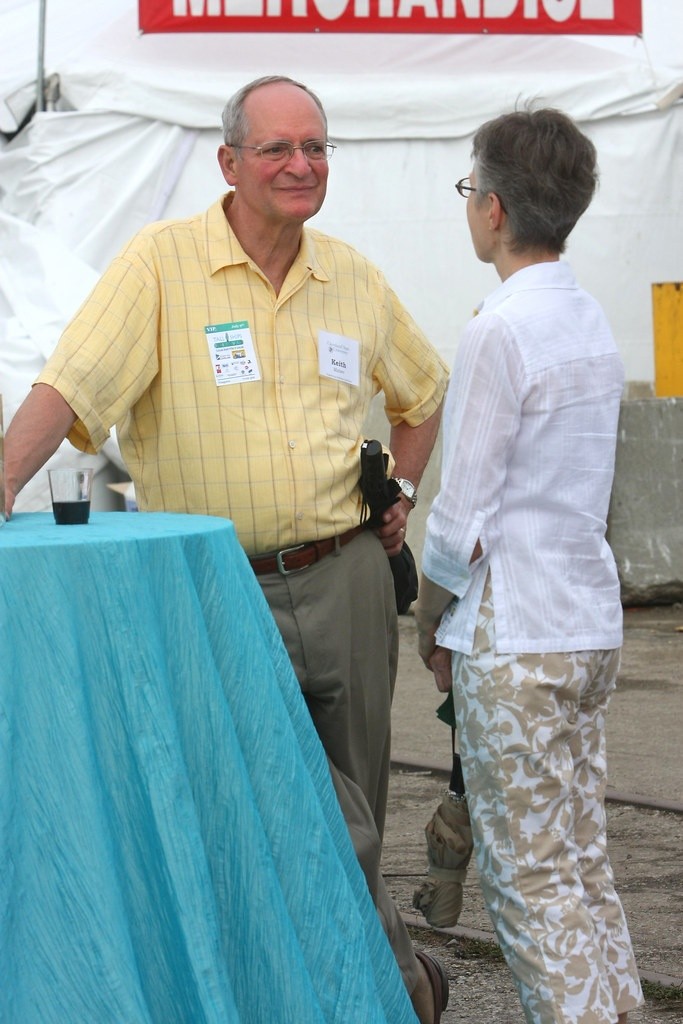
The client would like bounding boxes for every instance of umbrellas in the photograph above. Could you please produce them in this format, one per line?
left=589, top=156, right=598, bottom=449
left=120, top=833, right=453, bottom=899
left=358, top=438, right=420, bottom=617
left=416, top=753, right=476, bottom=928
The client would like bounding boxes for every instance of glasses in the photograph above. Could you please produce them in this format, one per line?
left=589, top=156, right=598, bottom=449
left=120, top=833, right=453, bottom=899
left=226, top=140, right=337, bottom=164
left=455, top=177, right=507, bottom=214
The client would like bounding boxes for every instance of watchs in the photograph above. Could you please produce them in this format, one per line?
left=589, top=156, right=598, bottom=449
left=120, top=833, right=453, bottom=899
left=390, top=475, right=418, bottom=509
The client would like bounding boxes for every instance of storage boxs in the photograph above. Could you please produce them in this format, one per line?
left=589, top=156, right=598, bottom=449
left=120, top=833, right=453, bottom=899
left=106, top=481, right=138, bottom=512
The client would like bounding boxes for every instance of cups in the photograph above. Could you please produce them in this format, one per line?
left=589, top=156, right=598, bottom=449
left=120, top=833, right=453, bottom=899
left=47, top=468, right=91, bottom=525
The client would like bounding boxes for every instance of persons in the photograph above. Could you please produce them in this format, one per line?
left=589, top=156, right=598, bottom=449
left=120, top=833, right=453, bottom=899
left=416, top=95, right=650, bottom=1024
left=0, top=73, right=455, bottom=1024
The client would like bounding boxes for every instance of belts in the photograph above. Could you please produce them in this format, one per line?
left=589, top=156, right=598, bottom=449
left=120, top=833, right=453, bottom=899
left=250, top=522, right=368, bottom=577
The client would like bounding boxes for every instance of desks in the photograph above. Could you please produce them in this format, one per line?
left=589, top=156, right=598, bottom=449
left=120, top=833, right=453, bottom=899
left=0, top=511, right=426, bottom=1024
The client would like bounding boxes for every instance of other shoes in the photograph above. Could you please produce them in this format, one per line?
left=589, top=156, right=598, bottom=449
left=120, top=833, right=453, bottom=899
left=414, top=951, right=449, bottom=1024
